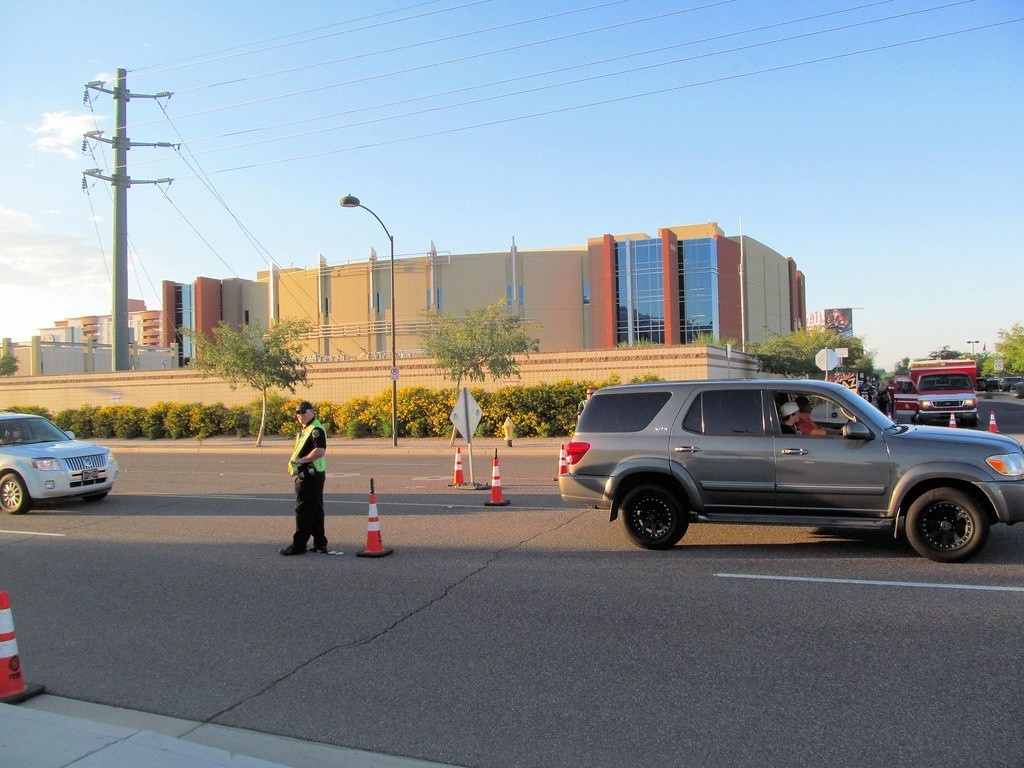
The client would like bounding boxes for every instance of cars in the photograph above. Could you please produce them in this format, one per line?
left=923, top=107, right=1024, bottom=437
left=976, top=378, right=987, bottom=391
left=858, top=381, right=876, bottom=395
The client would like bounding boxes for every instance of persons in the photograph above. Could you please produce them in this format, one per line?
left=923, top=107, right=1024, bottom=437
left=779, top=402, right=802, bottom=435
left=793, top=396, right=826, bottom=435
left=858, top=379, right=873, bottom=403
left=278, top=402, right=328, bottom=556
left=876, top=383, right=892, bottom=415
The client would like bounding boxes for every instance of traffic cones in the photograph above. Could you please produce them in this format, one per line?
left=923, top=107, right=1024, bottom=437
left=448, top=447, right=464, bottom=486
left=484, top=448, right=511, bottom=506
left=356, top=478, right=394, bottom=558
left=888, top=412, right=892, bottom=420
left=989, top=411, right=999, bottom=433
left=0, top=589, right=46, bottom=705
left=949, top=412, right=956, bottom=428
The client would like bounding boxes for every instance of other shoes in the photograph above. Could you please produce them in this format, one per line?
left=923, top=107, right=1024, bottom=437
left=309, top=544, right=328, bottom=554
left=278, top=545, right=306, bottom=556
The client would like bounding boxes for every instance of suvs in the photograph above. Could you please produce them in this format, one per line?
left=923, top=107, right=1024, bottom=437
left=0, top=408, right=119, bottom=515
left=558, top=380, right=1024, bottom=563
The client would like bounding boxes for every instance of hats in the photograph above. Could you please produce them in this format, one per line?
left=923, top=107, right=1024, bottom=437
left=296, top=402, right=312, bottom=412
left=779, top=402, right=800, bottom=419
left=795, top=396, right=809, bottom=407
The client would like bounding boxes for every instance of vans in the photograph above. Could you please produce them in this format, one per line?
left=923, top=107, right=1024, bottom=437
left=1000, top=377, right=1023, bottom=391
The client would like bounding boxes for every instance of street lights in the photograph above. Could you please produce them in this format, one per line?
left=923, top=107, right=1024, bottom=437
left=340, top=193, right=398, bottom=447
left=967, top=341, right=979, bottom=354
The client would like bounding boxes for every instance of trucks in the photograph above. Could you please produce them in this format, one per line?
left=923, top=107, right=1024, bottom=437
left=894, top=360, right=979, bottom=429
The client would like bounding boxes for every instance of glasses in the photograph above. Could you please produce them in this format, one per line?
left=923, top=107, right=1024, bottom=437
left=587, top=393, right=593, bottom=395
left=296, top=409, right=311, bottom=415
